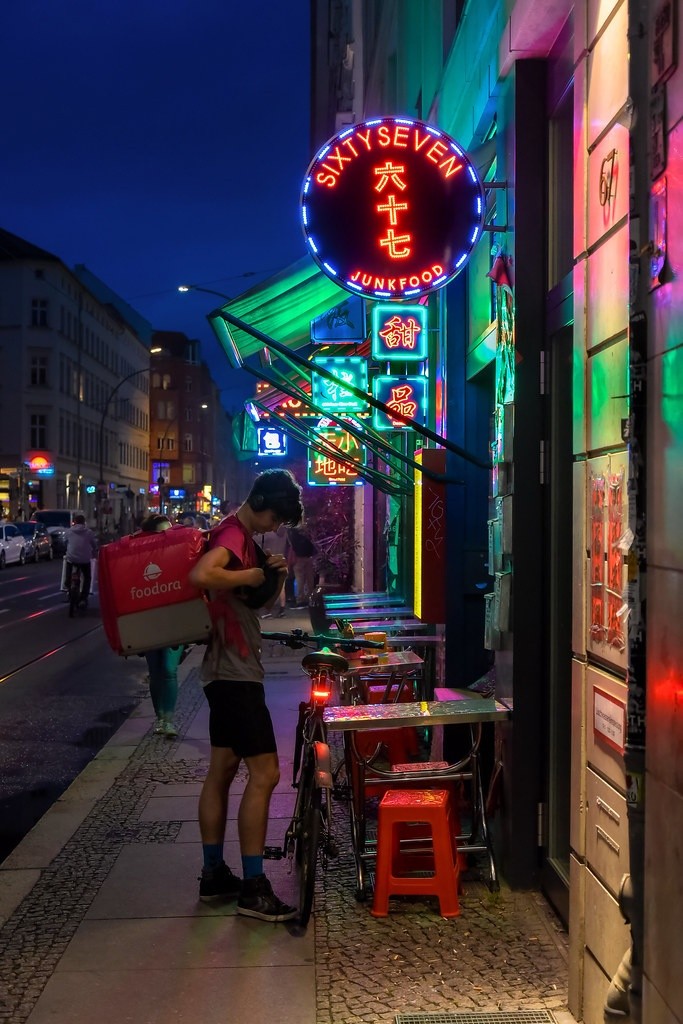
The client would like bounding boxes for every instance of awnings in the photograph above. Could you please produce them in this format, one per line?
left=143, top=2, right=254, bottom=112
left=207, top=250, right=490, bottom=485
left=240, top=304, right=415, bottom=497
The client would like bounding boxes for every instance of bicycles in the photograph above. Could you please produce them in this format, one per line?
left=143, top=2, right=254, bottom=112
left=260, top=628, right=385, bottom=936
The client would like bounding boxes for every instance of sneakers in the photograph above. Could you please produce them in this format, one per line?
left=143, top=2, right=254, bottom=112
left=153, top=718, right=167, bottom=734
left=235, top=874, right=297, bottom=921
left=196, top=865, right=241, bottom=901
left=165, top=722, right=178, bottom=737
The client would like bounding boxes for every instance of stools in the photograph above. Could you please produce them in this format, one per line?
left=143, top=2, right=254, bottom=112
left=330, top=622, right=468, bottom=917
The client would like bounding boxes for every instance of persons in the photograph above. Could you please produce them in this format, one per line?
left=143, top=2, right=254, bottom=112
left=188, top=470, right=298, bottom=924
left=64, top=503, right=313, bottom=738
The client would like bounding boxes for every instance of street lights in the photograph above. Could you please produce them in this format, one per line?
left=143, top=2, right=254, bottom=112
left=160, top=403, right=208, bottom=515
left=98, top=365, right=158, bottom=483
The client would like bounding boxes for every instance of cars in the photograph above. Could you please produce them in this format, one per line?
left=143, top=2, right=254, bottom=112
left=13, top=521, right=54, bottom=564
left=0, top=525, right=27, bottom=570
left=29, top=509, right=86, bottom=559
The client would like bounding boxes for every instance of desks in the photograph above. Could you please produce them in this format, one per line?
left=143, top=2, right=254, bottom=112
left=322, top=587, right=444, bottom=767
left=321, top=695, right=512, bottom=901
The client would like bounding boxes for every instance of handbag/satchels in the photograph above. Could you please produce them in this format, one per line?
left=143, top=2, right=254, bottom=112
left=231, top=534, right=282, bottom=611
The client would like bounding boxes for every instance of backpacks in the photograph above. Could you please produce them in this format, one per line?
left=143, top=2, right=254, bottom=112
left=97, top=516, right=257, bottom=658
